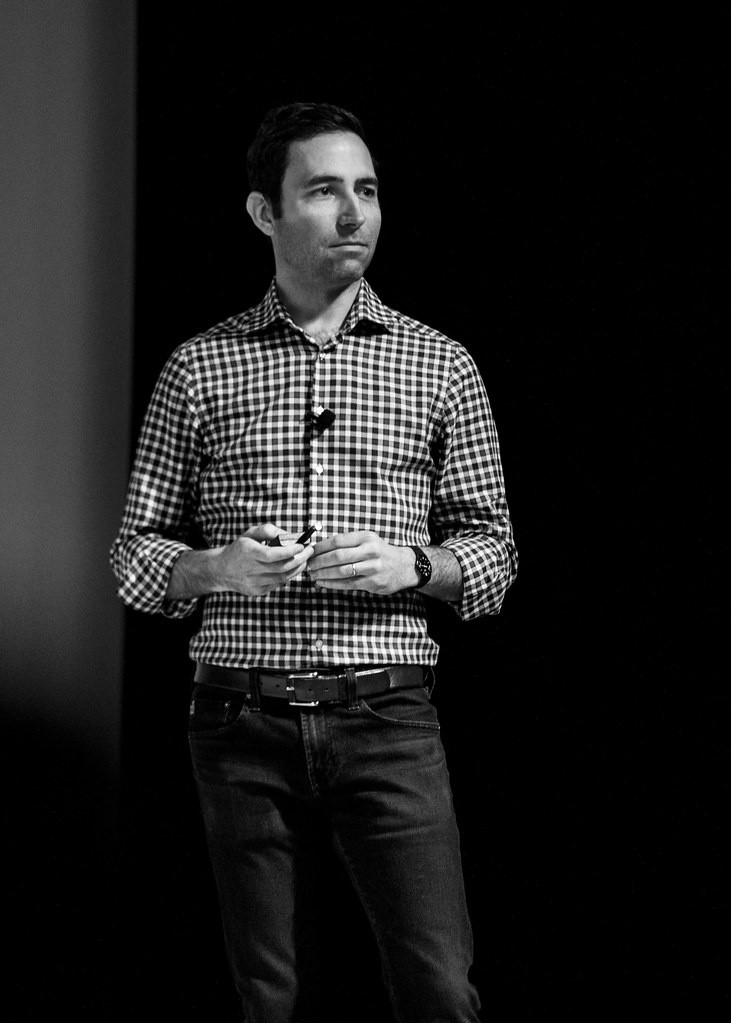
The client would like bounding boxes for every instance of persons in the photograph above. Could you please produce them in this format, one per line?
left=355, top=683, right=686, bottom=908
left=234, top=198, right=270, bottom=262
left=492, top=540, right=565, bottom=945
left=113, top=102, right=519, bottom=1022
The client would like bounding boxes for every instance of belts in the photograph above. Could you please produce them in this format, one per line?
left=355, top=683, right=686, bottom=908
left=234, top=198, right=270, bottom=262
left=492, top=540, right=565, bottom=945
left=193, top=662, right=430, bottom=708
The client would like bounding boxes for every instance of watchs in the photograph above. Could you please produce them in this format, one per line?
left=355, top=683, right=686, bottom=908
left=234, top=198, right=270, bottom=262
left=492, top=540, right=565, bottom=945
left=409, top=546, right=433, bottom=589
